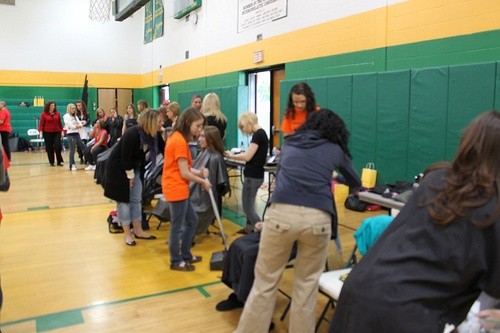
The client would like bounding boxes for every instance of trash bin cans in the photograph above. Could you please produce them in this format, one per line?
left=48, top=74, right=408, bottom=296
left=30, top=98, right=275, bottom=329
left=8, top=132, right=19, bottom=152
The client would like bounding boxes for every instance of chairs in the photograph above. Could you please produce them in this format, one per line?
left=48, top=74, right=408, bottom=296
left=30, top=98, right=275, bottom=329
left=314, top=214, right=394, bottom=333
left=27, top=130, right=44, bottom=151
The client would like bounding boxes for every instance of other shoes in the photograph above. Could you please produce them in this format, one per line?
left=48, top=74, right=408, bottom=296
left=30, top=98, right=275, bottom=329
left=85, top=164, right=96, bottom=171
left=51, top=163, right=55, bottom=167
left=71, top=165, right=77, bottom=171
left=134, top=232, right=156, bottom=239
left=185, top=256, right=201, bottom=263
left=80, top=159, right=84, bottom=164
left=236, top=228, right=253, bottom=234
left=125, top=236, right=136, bottom=246
left=60, top=162, right=63, bottom=166
left=215, top=293, right=242, bottom=311
left=170, top=262, right=194, bottom=271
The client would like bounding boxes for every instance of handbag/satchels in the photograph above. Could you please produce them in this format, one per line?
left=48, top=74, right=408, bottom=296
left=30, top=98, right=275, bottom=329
left=345, top=195, right=366, bottom=211
left=361, top=163, right=379, bottom=188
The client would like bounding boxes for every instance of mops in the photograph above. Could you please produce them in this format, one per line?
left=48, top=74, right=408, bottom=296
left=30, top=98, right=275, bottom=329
left=203, top=176, right=229, bottom=271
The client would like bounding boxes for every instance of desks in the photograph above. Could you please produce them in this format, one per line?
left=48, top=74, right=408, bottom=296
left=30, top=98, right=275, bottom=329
left=223, top=150, right=277, bottom=222
left=358, top=191, right=407, bottom=212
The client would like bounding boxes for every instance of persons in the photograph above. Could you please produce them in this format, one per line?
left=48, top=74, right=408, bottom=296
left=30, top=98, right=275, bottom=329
left=63, top=103, right=85, bottom=170
left=97, top=108, right=111, bottom=121
left=0, top=101, right=12, bottom=162
left=105, top=108, right=123, bottom=142
left=138, top=100, right=148, bottom=111
left=236, top=108, right=360, bottom=333
left=161, top=107, right=212, bottom=272
left=83, top=119, right=108, bottom=170
left=76, top=101, right=91, bottom=164
left=160, top=102, right=179, bottom=142
left=216, top=222, right=298, bottom=311
left=39, top=102, right=64, bottom=166
left=102, top=109, right=165, bottom=246
left=155, top=126, right=232, bottom=233
left=279, top=83, right=321, bottom=135
left=326, top=110, right=500, bottom=333
left=191, top=95, right=202, bottom=109
left=202, top=92, right=227, bottom=138
left=122, top=104, right=139, bottom=135
left=224, top=112, right=268, bottom=234
